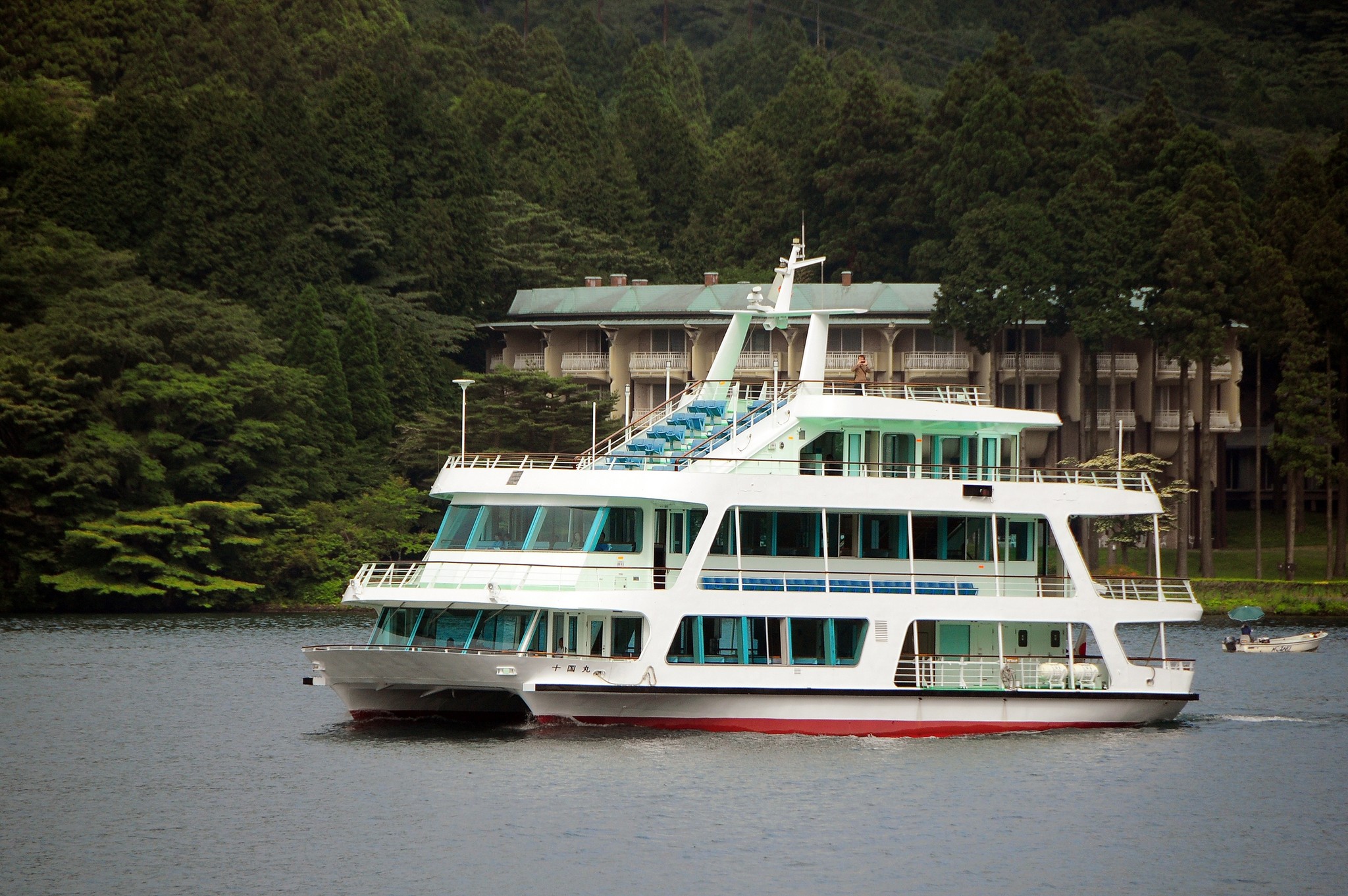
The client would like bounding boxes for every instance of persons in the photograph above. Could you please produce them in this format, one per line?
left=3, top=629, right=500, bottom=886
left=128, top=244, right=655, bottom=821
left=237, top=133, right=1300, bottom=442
left=1241, top=622, right=1251, bottom=635
left=553, top=637, right=563, bottom=658
left=851, top=354, right=871, bottom=395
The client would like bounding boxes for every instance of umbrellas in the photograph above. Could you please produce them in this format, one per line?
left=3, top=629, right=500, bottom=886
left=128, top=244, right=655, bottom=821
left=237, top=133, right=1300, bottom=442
left=1227, top=605, right=1265, bottom=623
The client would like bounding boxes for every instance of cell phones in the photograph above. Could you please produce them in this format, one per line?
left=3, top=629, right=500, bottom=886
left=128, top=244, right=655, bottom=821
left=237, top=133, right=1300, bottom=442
left=861, top=361, right=865, bottom=363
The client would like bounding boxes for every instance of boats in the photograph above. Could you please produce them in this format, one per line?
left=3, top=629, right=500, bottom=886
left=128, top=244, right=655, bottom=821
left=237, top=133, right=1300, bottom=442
left=301, top=207, right=1205, bottom=736
left=1221, top=630, right=1328, bottom=653
